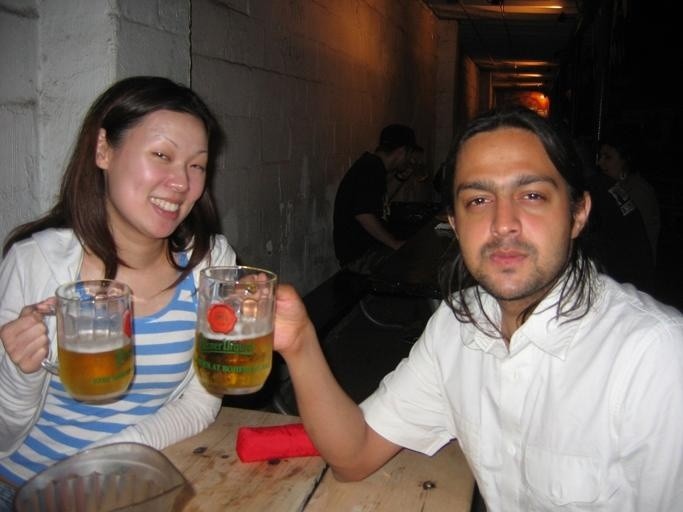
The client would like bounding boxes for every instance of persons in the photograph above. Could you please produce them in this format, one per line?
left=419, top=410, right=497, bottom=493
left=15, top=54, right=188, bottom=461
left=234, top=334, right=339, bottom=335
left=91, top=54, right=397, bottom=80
left=1, top=75, right=238, bottom=506
left=334, top=122, right=661, bottom=346
left=225, top=103, right=682, bottom=512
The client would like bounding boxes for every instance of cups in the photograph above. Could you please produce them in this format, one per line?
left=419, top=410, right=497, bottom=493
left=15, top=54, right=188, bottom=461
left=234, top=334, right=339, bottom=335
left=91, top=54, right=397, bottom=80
left=29, top=277, right=135, bottom=404
left=193, top=264, right=279, bottom=400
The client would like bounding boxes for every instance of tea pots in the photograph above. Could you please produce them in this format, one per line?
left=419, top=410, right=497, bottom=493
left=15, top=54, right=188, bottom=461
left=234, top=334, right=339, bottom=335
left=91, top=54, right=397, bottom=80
left=0, top=441, right=186, bottom=512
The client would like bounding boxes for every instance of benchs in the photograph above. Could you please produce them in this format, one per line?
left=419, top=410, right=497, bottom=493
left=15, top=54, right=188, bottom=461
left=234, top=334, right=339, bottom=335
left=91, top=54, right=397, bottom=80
left=303, top=268, right=370, bottom=341
